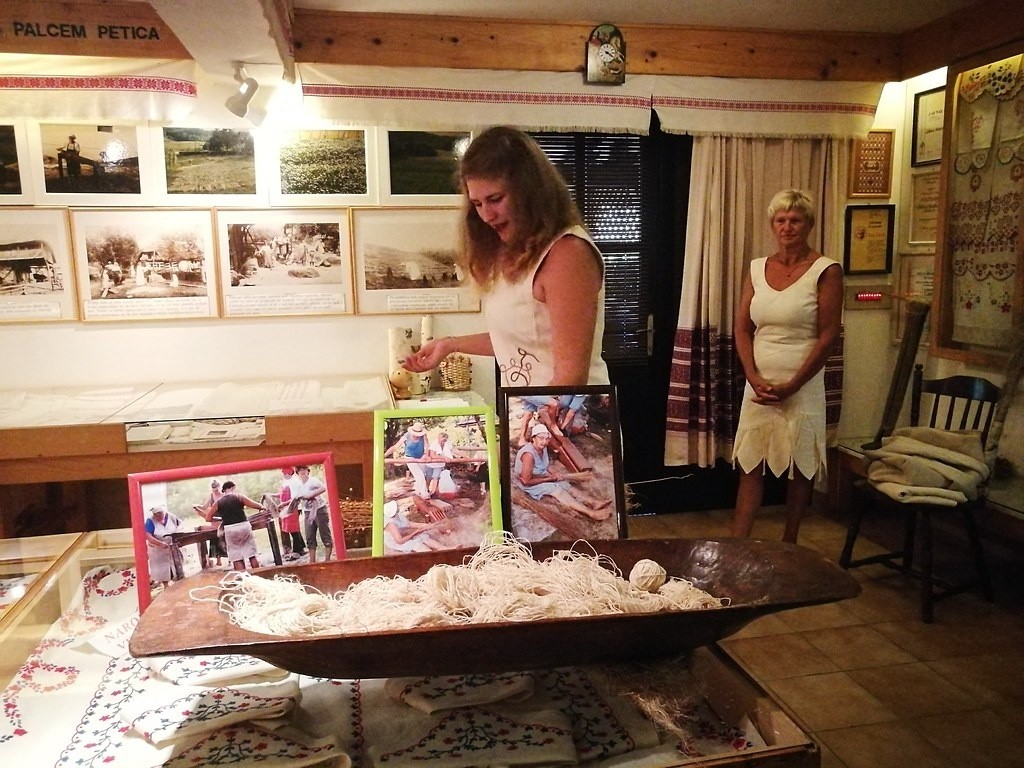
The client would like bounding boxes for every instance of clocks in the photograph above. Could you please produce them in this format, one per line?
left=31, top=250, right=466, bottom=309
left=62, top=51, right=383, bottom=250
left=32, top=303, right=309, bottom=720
left=586, top=23, right=626, bottom=84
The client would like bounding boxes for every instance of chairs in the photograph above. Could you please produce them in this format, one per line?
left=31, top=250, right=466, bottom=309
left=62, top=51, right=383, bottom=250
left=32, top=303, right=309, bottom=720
left=837, top=362, right=1004, bottom=626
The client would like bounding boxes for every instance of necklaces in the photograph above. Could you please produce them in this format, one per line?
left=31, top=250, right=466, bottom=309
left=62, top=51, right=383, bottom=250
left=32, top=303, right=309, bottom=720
left=786, top=260, right=802, bottom=278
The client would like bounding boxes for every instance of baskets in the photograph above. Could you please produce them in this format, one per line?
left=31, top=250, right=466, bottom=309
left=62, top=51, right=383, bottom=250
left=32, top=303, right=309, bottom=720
left=439, top=355, right=471, bottom=391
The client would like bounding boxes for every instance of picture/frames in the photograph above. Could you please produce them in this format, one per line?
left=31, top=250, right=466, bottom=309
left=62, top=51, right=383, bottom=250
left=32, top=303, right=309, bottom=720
left=841, top=84, right=946, bottom=348
left=0, top=203, right=482, bottom=326
left=127, top=453, right=345, bottom=621
left=370, top=403, right=502, bottom=556
left=498, top=384, right=627, bottom=544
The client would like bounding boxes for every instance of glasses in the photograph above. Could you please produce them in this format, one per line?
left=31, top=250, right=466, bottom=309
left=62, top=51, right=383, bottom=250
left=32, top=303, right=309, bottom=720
left=537, top=436, right=551, bottom=441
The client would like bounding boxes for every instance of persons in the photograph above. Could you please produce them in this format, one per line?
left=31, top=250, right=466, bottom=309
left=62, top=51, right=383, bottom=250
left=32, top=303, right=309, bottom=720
left=560, top=395, right=589, bottom=433
left=77, top=210, right=466, bottom=295
left=143, top=464, right=333, bottom=592
left=398, top=124, right=625, bottom=463
left=516, top=396, right=563, bottom=448
left=513, top=425, right=612, bottom=520
left=56, top=135, right=81, bottom=177
left=385, top=422, right=432, bottom=499
left=425, top=433, right=473, bottom=496
left=731, top=188, right=841, bottom=550
left=383, top=499, right=450, bottom=554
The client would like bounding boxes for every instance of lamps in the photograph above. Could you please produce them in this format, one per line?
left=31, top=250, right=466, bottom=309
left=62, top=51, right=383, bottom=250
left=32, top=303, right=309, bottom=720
left=223, top=60, right=259, bottom=119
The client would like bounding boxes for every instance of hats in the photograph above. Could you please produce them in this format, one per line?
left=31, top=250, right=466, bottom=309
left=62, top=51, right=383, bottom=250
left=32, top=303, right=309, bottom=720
left=384, top=500, right=397, bottom=517
left=407, top=423, right=427, bottom=436
left=531, top=424, right=551, bottom=438
left=212, top=480, right=219, bottom=488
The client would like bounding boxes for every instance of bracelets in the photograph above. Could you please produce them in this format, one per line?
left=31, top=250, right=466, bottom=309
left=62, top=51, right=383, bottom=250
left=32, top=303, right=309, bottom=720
left=445, top=336, right=456, bottom=358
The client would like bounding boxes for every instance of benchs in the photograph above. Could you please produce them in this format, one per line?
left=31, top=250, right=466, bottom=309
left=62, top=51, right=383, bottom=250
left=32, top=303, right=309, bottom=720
left=825, top=432, right=1023, bottom=616
